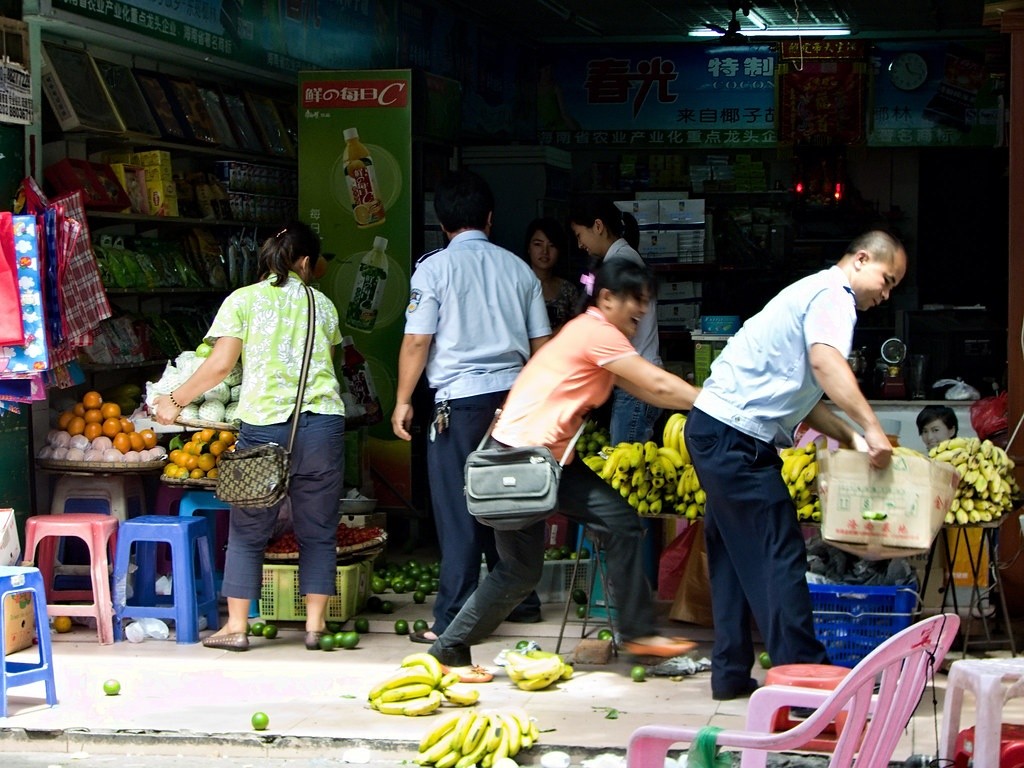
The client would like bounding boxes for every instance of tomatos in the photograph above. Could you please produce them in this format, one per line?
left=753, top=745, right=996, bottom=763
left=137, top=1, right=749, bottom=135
left=266, top=522, right=380, bottom=553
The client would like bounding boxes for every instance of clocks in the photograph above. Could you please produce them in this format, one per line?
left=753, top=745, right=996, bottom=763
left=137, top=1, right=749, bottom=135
left=886, top=51, right=928, bottom=91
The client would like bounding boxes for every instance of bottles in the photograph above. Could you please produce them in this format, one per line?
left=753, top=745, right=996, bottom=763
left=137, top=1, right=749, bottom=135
left=341, top=336, right=384, bottom=427
left=344, top=236, right=389, bottom=334
left=344, top=127, right=386, bottom=229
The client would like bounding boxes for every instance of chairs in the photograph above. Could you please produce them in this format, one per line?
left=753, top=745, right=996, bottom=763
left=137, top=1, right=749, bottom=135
left=628, top=613, right=961, bottom=768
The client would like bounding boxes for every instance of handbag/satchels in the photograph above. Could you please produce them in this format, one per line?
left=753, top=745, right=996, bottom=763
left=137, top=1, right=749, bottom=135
left=461, top=441, right=565, bottom=523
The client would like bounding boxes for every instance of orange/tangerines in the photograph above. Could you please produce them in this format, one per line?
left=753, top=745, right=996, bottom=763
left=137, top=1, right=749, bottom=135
left=39, top=390, right=167, bottom=463
left=54, top=544, right=775, bottom=728
left=162, top=428, right=238, bottom=479
left=576, top=420, right=610, bottom=460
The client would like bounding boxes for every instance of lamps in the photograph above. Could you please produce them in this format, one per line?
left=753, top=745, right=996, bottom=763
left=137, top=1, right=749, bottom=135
left=686, top=28, right=857, bottom=38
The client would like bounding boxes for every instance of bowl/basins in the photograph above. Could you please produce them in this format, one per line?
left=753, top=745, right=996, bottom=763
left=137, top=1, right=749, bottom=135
left=338, top=498, right=378, bottom=514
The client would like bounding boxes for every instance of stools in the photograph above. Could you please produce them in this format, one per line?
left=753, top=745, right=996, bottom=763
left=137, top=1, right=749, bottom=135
left=110, top=514, right=221, bottom=644
left=556, top=523, right=648, bottom=654
left=0, top=565, right=58, bottom=719
left=764, top=665, right=868, bottom=752
left=171, top=491, right=260, bottom=617
left=50, top=471, right=148, bottom=590
left=24, top=513, right=119, bottom=646
left=939, top=658, right=1024, bottom=768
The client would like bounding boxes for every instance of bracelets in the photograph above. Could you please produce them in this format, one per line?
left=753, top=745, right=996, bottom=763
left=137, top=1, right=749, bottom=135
left=170, top=391, right=185, bottom=408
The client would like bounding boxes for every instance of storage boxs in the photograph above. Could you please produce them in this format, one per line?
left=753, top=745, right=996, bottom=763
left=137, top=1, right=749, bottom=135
left=813, top=436, right=962, bottom=561
left=0, top=508, right=21, bottom=565
left=4, top=560, right=33, bottom=656
left=477, top=558, right=591, bottom=604
left=613, top=155, right=768, bottom=326
left=259, top=553, right=377, bottom=622
left=806, top=582, right=913, bottom=669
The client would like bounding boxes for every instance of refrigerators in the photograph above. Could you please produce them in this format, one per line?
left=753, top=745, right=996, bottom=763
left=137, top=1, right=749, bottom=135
left=294, top=69, right=464, bottom=553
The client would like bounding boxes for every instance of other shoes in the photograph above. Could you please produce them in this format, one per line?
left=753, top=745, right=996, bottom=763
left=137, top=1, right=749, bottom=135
left=712, top=678, right=757, bottom=700
left=203, top=632, right=249, bottom=651
left=304, top=631, right=333, bottom=650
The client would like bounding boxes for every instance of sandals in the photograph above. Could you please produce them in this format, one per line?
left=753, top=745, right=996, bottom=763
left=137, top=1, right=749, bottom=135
left=503, top=610, right=542, bottom=622
left=408, top=629, right=437, bottom=644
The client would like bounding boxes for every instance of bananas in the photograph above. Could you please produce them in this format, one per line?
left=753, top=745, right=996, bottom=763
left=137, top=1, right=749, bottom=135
left=928, top=436, right=1020, bottom=525
left=778, top=442, right=824, bottom=523
left=367, top=646, right=573, bottom=768
left=581, top=414, right=707, bottom=520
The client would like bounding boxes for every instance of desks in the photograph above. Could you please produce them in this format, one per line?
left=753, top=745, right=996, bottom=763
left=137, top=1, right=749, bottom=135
left=639, top=507, right=1016, bottom=660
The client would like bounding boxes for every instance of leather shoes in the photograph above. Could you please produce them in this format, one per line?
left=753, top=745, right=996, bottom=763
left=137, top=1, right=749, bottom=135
left=440, top=662, right=493, bottom=682
left=624, top=635, right=699, bottom=657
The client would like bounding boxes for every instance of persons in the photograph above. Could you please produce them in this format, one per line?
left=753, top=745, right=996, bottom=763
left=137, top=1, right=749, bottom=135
left=391, top=177, right=543, bottom=645
left=916, top=405, right=958, bottom=455
left=569, top=192, right=664, bottom=592
left=430, top=258, right=701, bottom=683
left=684, top=230, right=906, bottom=703
left=521, top=227, right=579, bottom=338
left=152, top=220, right=345, bottom=649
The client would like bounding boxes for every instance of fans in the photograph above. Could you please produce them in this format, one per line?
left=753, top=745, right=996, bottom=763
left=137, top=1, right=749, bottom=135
left=687, top=6, right=783, bottom=46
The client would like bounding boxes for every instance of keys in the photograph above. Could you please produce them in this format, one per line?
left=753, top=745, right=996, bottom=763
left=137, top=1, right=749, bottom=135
left=437, top=414, right=449, bottom=434
